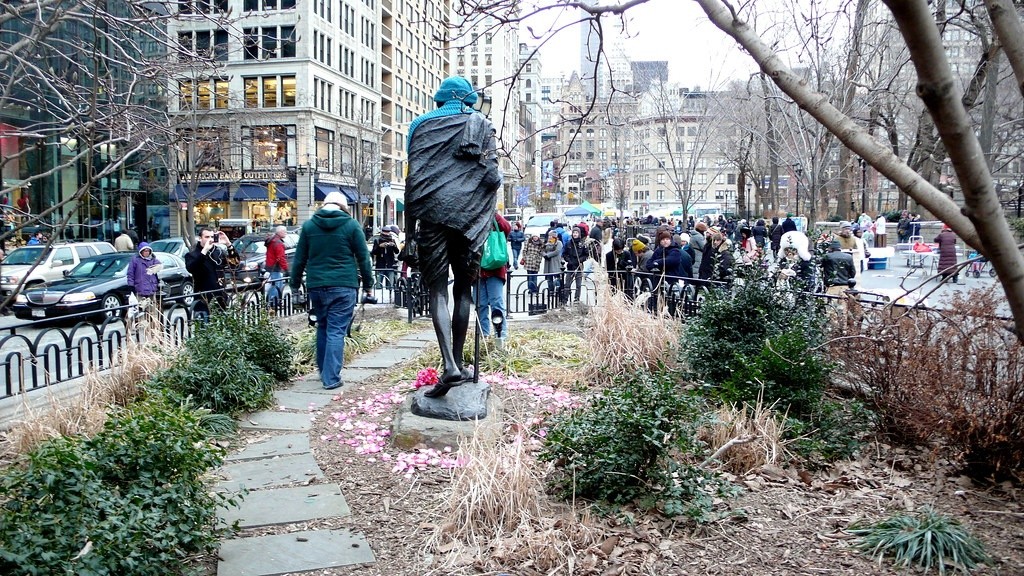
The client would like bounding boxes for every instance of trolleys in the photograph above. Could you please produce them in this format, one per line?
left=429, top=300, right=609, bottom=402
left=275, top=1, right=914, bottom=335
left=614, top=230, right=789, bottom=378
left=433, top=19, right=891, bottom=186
left=964, top=249, right=997, bottom=278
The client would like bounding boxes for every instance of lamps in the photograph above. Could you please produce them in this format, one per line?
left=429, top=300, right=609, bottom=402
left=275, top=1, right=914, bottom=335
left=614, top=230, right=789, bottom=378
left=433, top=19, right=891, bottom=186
left=307, top=307, right=318, bottom=327
left=491, top=310, right=504, bottom=338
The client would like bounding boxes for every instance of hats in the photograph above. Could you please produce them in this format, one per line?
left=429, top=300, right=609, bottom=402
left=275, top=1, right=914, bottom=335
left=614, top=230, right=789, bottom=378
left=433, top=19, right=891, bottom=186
left=786, top=212, right=794, bottom=217
left=711, top=232, right=726, bottom=249
left=531, top=231, right=540, bottom=239
left=839, top=220, right=851, bottom=230
left=680, top=233, right=690, bottom=245
left=778, top=230, right=811, bottom=261
left=696, top=222, right=707, bottom=231
left=382, top=226, right=392, bottom=231
left=660, top=230, right=671, bottom=242
left=740, top=226, right=751, bottom=239
left=323, top=191, right=349, bottom=212
left=433, top=77, right=478, bottom=104
left=632, top=239, right=645, bottom=251
left=572, top=227, right=580, bottom=234
left=391, top=224, right=400, bottom=234
left=830, top=240, right=842, bottom=251
left=942, top=224, right=951, bottom=231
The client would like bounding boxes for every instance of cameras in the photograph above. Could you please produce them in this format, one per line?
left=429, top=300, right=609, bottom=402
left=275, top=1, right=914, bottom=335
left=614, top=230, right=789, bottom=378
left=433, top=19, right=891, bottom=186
left=361, top=289, right=378, bottom=304
left=385, top=243, right=391, bottom=246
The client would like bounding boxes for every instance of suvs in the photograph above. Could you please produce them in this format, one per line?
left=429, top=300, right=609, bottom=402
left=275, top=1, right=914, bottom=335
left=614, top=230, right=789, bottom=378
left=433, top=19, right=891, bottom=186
left=0, top=240, right=120, bottom=300
left=523, top=211, right=570, bottom=242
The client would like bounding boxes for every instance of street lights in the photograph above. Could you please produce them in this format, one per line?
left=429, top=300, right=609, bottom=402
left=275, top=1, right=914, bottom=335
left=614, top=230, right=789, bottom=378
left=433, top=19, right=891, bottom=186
left=792, top=162, right=803, bottom=217
left=725, top=188, right=729, bottom=219
left=99, top=134, right=120, bottom=241
left=745, top=181, right=753, bottom=220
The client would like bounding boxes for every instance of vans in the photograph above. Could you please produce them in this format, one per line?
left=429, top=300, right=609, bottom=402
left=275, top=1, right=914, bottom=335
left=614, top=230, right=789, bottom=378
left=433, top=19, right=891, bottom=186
left=502, top=214, right=522, bottom=228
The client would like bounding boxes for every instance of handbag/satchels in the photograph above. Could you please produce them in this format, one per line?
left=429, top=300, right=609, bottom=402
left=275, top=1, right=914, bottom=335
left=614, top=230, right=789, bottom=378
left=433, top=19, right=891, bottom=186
left=481, top=218, right=507, bottom=270
left=397, top=248, right=404, bottom=261
left=128, top=291, right=138, bottom=318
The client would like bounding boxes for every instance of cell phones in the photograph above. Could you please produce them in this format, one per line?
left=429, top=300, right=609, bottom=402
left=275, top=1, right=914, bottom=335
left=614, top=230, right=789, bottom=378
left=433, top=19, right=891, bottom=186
left=214, top=233, right=219, bottom=242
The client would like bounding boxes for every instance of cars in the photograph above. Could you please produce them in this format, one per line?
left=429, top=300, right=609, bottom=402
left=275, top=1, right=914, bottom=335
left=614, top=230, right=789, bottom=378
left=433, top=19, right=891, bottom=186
left=229, top=233, right=301, bottom=281
left=364, top=231, right=405, bottom=252
left=13, top=249, right=195, bottom=323
left=144, top=237, right=201, bottom=258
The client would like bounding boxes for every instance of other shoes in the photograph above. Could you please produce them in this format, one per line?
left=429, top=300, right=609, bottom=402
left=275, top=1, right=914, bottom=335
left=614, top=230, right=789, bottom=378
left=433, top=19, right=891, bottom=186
left=322, top=375, right=343, bottom=390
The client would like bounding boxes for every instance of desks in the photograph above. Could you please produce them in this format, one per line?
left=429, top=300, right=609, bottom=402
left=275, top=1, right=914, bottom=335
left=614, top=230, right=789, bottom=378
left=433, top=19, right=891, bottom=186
left=902, top=249, right=964, bottom=282
left=866, top=246, right=896, bottom=269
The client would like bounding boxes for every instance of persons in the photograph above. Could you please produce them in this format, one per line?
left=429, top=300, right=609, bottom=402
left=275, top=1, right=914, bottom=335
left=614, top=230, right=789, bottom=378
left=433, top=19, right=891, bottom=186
left=934, top=224, right=959, bottom=283
left=403, top=75, right=503, bottom=398
left=264, top=225, right=289, bottom=310
left=114, top=230, right=134, bottom=252
left=507, top=212, right=920, bottom=317
left=127, top=242, right=163, bottom=305
left=397, top=239, right=419, bottom=281
left=27, top=231, right=48, bottom=245
left=184, top=226, right=240, bottom=330
left=473, top=211, right=511, bottom=344
left=126, top=224, right=139, bottom=249
left=290, top=191, right=374, bottom=390
left=370, top=226, right=399, bottom=289
left=151, top=225, right=161, bottom=241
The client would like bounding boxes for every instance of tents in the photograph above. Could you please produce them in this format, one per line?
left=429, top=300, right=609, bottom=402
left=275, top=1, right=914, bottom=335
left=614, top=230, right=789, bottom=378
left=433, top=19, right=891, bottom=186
left=562, top=199, right=602, bottom=219
